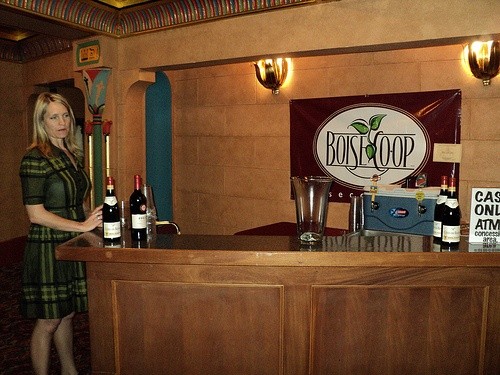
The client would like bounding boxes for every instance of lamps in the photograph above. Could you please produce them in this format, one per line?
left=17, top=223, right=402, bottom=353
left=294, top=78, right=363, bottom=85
left=252, top=57, right=288, bottom=95
left=467, top=40, right=500, bottom=86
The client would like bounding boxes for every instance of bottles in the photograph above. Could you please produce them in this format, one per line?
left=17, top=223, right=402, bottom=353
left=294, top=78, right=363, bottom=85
left=129, top=175, right=147, bottom=240
left=102, top=176, right=123, bottom=241
left=432, top=175, right=449, bottom=241
left=441, top=178, right=461, bottom=246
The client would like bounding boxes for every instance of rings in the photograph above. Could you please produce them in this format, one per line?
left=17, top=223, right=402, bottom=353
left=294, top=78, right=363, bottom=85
left=97, top=217, right=99, bottom=220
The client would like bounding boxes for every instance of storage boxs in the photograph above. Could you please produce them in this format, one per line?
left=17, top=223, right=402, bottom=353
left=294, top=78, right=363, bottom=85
left=362, top=186, right=440, bottom=236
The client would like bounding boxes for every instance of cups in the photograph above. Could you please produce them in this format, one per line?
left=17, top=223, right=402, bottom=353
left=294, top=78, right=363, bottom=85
left=290, top=176, right=332, bottom=245
left=141, top=184, right=157, bottom=235
left=118, top=201, right=127, bottom=232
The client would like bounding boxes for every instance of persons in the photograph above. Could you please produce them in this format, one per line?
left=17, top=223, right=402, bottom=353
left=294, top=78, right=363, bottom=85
left=19, top=93, right=103, bottom=375
